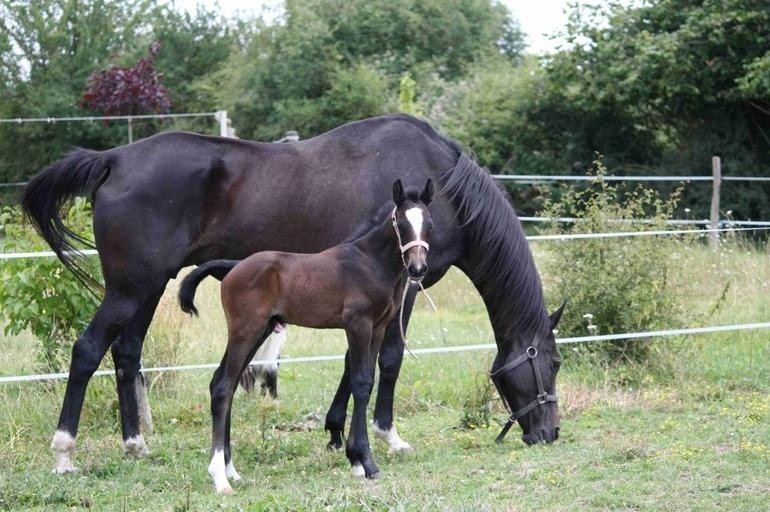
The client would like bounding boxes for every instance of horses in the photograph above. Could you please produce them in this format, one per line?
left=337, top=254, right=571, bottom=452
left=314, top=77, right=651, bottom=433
left=20, top=109, right=570, bottom=479
left=177, top=178, right=436, bottom=499
